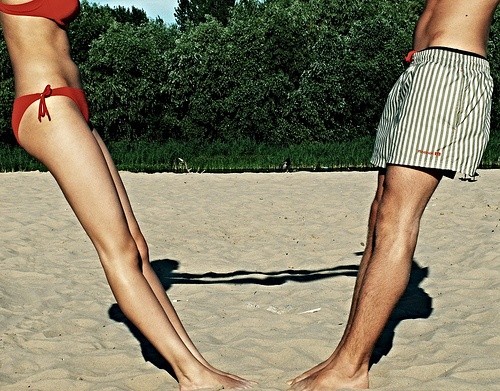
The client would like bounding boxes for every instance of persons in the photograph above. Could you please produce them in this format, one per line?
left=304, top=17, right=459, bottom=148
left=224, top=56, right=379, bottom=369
left=1, top=1, right=261, bottom=391
left=287, top=0, right=498, bottom=388
